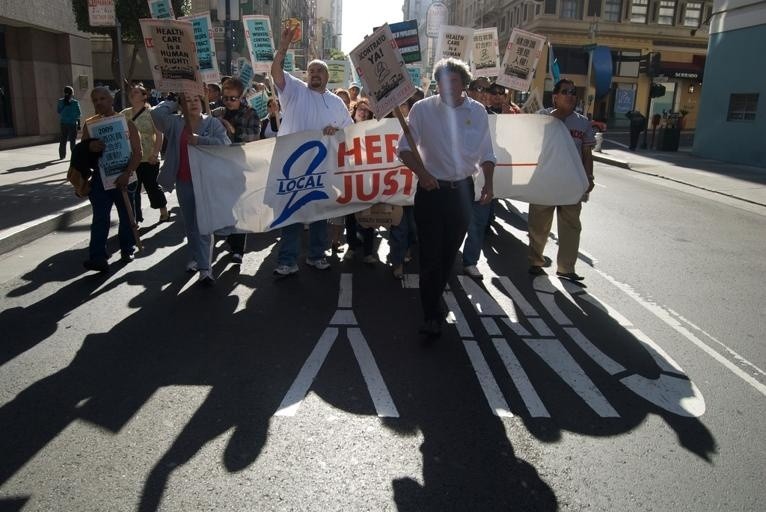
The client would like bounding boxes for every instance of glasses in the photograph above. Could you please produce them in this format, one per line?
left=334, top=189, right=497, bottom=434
left=560, top=88, right=576, bottom=95
left=475, top=86, right=485, bottom=92
left=491, top=90, right=505, bottom=95
left=223, top=96, right=238, bottom=101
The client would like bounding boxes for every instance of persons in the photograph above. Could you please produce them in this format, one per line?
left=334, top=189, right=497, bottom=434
left=504, top=88, right=521, bottom=114
left=261, top=99, right=282, bottom=140
left=412, top=86, right=424, bottom=100
left=56, top=86, right=81, bottom=160
left=625, top=107, right=646, bottom=152
left=271, top=20, right=355, bottom=279
left=528, top=80, right=595, bottom=281
left=205, top=77, right=261, bottom=264
left=327, top=88, right=350, bottom=252
left=459, top=75, right=499, bottom=280
left=206, top=82, right=222, bottom=109
left=488, top=83, right=515, bottom=114
left=80, top=87, right=142, bottom=271
left=342, top=100, right=382, bottom=267
left=118, top=83, right=171, bottom=237
left=348, top=83, right=361, bottom=107
left=396, top=58, right=496, bottom=338
left=388, top=97, right=416, bottom=281
left=149, top=93, right=232, bottom=288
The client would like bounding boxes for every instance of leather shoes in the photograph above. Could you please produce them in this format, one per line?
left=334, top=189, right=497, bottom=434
left=83, top=257, right=109, bottom=271
left=121, top=250, right=134, bottom=261
left=418, top=319, right=441, bottom=338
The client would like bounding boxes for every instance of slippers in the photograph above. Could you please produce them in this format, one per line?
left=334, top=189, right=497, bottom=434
left=160, top=210, right=171, bottom=223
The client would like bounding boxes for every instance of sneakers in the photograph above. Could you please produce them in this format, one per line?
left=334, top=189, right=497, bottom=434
left=305, top=256, right=330, bottom=270
left=186, top=259, right=198, bottom=272
left=465, top=265, right=483, bottom=278
left=363, top=255, right=377, bottom=263
left=528, top=265, right=543, bottom=275
left=230, top=253, right=243, bottom=263
left=343, top=250, right=354, bottom=261
left=272, top=263, right=299, bottom=281
left=199, top=270, right=215, bottom=282
left=393, top=263, right=404, bottom=280
left=555, top=271, right=585, bottom=283
